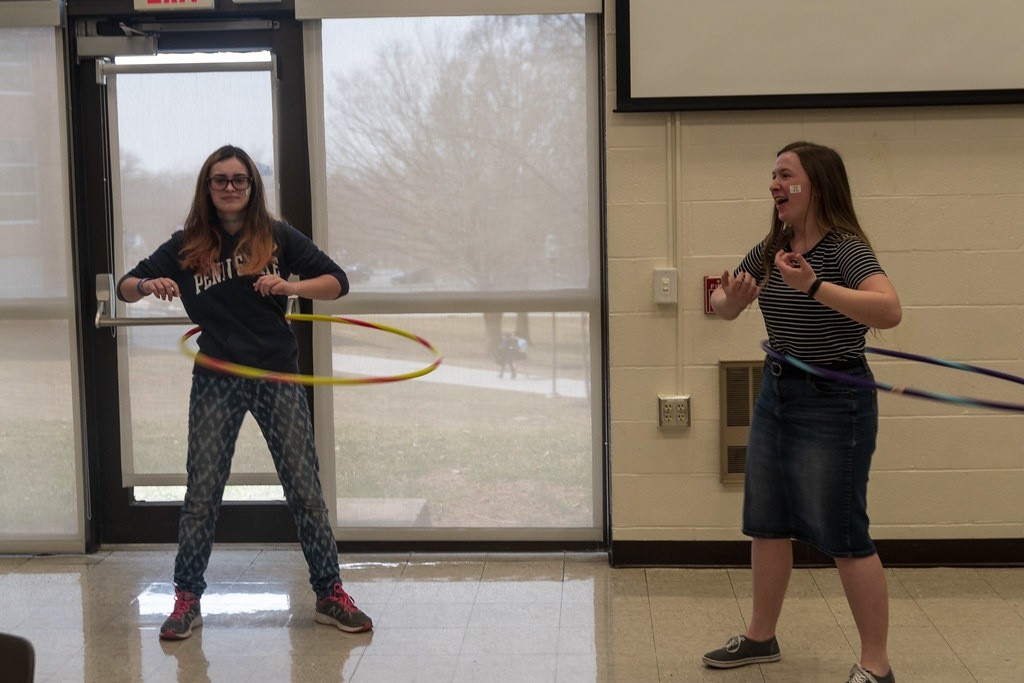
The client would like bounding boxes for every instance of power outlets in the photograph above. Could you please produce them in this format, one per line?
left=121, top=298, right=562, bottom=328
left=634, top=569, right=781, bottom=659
left=659, top=394, right=692, bottom=428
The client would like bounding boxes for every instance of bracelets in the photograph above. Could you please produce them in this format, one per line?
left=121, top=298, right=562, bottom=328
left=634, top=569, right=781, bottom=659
left=806, top=279, right=823, bottom=300
left=138, top=278, right=153, bottom=297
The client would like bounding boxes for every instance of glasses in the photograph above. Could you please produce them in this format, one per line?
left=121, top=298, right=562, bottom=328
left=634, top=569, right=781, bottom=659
left=206, top=177, right=252, bottom=191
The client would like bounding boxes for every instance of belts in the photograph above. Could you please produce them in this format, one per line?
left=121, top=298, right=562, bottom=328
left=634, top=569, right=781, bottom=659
left=764, top=356, right=868, bottom=378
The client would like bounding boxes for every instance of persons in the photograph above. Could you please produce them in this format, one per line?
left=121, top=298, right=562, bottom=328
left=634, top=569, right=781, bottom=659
left=116, top=145, right=373, bottom=641
left=498, top=332, right=529, bottom=379
left=701, top=143, right=902, bottom=683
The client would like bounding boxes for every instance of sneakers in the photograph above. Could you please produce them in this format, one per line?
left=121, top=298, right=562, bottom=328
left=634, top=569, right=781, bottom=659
left=159, top=591, right=203, bottom=639
left=315, top=583, right=373, bottom=633
left=701, top=632, right=782, bottom=669
left=847, top=661, right=895, bottom=683
